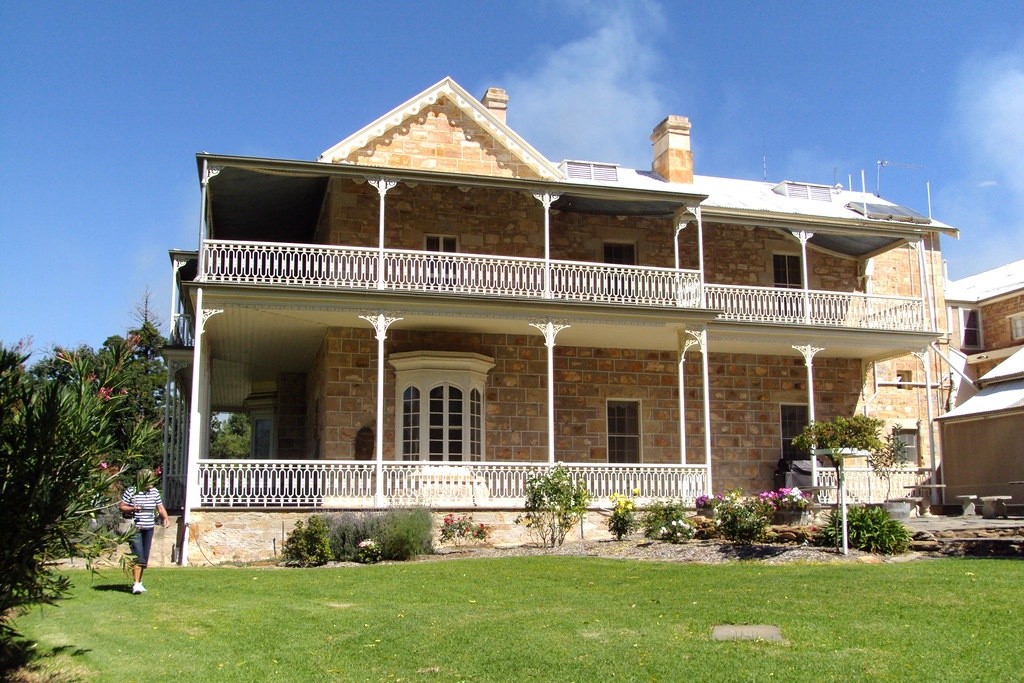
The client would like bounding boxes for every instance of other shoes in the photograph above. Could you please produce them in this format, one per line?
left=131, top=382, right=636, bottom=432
left=132, top=582, right=146, bottom=595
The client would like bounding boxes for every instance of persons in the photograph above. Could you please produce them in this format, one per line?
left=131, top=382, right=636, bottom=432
left=775, top=459, right=790, bottom=488
left=120, top=469, right=170, bottom=594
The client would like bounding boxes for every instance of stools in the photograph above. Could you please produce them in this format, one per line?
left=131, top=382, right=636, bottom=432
left=980, top=496, right=1012, bottom=519
left=903, top=497, right=922, bottom=517
left=956, top=495, right=977, bottom=516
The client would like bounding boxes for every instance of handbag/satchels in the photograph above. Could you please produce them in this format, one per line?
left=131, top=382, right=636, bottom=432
left=122, top=486, right=135, bottom=519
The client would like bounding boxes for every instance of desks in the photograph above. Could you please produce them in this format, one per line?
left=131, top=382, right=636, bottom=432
left=903, top=485, right=947, bottom=517
left=1008, top=481, right=1024, bottom=485
